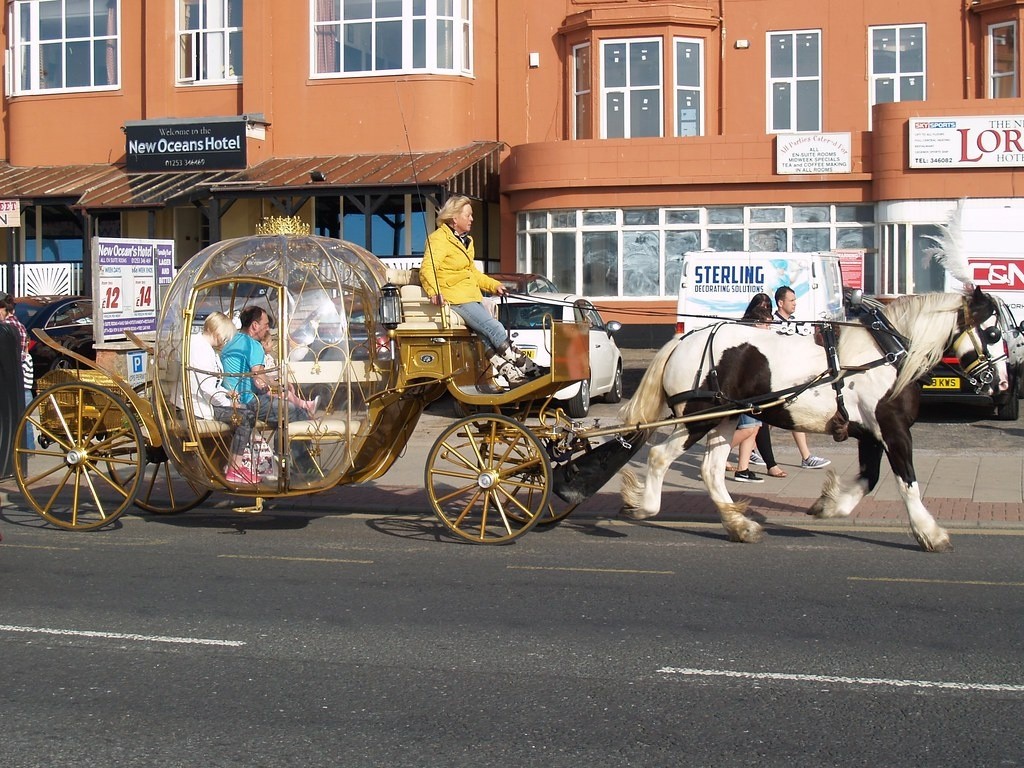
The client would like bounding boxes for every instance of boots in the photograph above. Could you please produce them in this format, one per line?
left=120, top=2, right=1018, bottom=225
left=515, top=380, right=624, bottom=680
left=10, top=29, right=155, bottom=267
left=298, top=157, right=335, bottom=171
left=484, top=347, right=536, bottom=390
left=496, top=337, right=550, bottom=381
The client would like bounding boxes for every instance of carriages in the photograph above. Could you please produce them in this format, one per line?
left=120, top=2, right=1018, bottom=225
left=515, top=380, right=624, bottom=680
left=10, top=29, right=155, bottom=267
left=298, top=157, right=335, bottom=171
left=22, top=215, right=1009, bottom=551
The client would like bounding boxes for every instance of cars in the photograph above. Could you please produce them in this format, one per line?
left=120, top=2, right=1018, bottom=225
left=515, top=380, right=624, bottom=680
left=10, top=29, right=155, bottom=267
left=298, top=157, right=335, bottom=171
left=192, top=284, right=279, bottom=329
left=920, top=296, right=1024, bottom=421
left=290, top=293, right=393, bottom=360
left=12, top=296, right=94, bottom=398
left=455, top=273, right=624, bottom=418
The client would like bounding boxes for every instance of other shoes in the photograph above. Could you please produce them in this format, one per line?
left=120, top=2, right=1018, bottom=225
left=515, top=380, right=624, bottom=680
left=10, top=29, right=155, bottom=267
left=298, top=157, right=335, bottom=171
left=768, top=469, right=787, bottom=477
left=302, top=395, right=322, bottom=416
left=726, top=466, right=738, bottom=471
left=269, top=452, right=299, bottom=478
left=294, top=462, right=329, bottom=477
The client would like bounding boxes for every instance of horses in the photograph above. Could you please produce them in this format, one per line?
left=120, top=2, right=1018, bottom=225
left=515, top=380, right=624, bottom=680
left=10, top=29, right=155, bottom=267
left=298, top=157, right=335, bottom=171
left=620, top=284, right=1011, bottom=550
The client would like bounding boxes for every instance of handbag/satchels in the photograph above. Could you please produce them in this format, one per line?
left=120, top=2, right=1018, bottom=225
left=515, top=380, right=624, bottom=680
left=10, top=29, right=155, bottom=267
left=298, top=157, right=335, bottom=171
left=223, top=426, right=279, bottom=481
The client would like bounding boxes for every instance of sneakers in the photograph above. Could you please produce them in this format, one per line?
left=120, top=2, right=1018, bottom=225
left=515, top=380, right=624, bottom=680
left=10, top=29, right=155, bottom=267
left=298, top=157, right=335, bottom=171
left=802, top=455, right=831, bottom=468
left=734, top=468, right=764, bottom=483
left=225, top=464, right=262, bottom=483
left=736, top=451, right=766, bottom=465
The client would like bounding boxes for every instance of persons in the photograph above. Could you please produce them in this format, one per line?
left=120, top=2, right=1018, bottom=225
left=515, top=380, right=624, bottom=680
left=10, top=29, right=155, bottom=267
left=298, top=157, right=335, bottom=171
left=749, top=286, right=831, bottom=468
left=258, top=331, right=322, bottom=415
left=169, top=311, right=261, bottom=483
left=222, top=306, right=329, bottom=475
left=419, top=195, right=550, bottom=389
left=0, top=290, right=36, bottom=459
left=725, top=293, right=787, bottom=483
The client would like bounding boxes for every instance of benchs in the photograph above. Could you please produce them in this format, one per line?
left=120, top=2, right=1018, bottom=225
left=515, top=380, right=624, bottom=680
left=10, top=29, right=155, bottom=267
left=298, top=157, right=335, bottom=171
left=169, top=411, right=277, bottom=475
left=284, top=420, right=361, bottom=477
left=384, top=263, right=498, bottom=390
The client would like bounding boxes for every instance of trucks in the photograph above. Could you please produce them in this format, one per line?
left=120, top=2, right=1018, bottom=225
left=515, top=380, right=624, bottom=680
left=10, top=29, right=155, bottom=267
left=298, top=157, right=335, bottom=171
left=675, top=248, right=864, bottom=335
